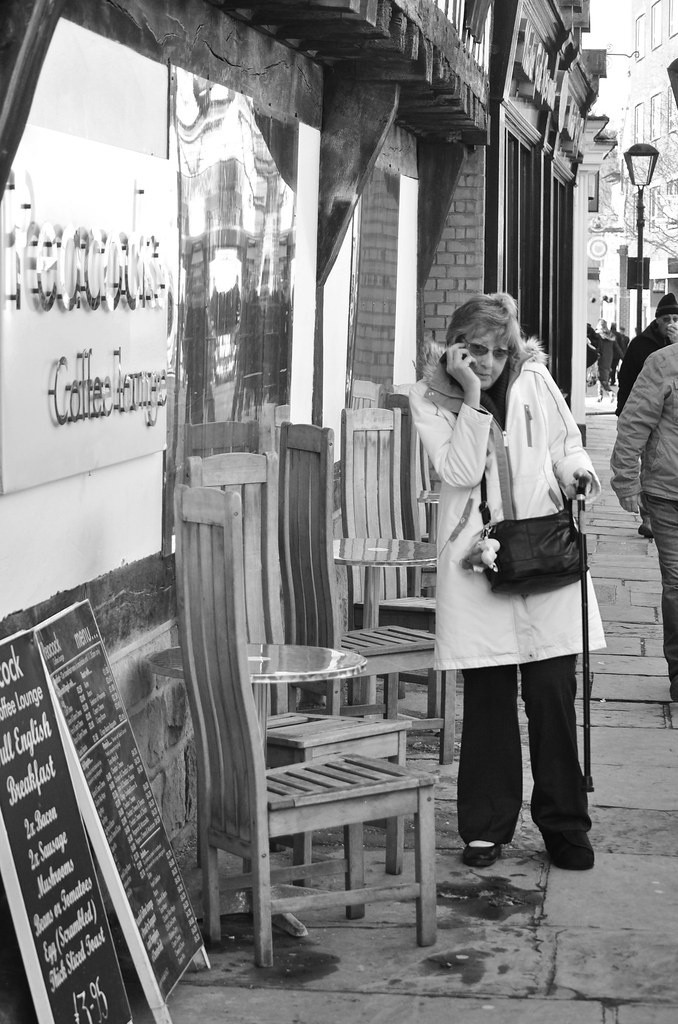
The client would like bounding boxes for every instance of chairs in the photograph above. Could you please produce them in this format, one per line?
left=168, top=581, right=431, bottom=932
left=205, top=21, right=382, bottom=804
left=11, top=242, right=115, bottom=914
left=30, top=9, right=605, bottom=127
left=340, top=404, right=444, bottom=721
left=170, top=481, right=442, bottom=971
left=347, top=377, right=441, bottom=607
left=278, top=420, right=458, bottom=766
left=181, top=449, right=417, bottom=891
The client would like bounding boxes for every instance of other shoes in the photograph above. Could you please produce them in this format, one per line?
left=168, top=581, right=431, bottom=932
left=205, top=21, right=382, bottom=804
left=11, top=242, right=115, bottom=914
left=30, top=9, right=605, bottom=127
left=610, top=384, right=616, bottom=386
left=464, top=841, right=500, bottom=866
left=546, top=835, right=595, bottom=870
left=671, top=682, right=678, bottom=701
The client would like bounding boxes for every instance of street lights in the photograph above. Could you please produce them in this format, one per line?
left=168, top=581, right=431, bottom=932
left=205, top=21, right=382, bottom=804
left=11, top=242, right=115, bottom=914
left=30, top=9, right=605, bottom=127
left=623, top=143, right=660, bottom=336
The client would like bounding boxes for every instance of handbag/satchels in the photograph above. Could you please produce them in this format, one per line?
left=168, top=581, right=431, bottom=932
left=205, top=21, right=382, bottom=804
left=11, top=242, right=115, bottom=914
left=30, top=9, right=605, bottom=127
left=483, top=510, right=589, bottom=596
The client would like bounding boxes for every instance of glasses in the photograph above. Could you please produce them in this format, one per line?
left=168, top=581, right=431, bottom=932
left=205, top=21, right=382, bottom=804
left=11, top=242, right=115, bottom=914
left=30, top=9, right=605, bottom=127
left=463, top=335, right=516, bottom=360
left=661, top=316, right=678, bottom=322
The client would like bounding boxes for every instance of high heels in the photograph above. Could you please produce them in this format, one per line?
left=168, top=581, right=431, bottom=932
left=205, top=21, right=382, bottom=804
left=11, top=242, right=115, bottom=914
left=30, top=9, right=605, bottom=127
left=609, top=391, right=616, bottom=403
left=597, top=394, right=603, bottom=402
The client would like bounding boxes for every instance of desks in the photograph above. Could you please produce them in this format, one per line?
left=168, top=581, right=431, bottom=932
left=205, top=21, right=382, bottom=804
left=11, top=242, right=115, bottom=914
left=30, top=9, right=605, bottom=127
left=329, top=536, right=441, bottom=720
left=142, top=642, right=370, bottom=938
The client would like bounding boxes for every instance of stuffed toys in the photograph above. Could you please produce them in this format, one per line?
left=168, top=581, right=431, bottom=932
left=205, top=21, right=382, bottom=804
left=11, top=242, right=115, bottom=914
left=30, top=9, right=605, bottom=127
left=469, top=538, right=486, bottom=564
left=478, top=538, right=499, bottom=569
left=459, top=553, right=476, bottom=574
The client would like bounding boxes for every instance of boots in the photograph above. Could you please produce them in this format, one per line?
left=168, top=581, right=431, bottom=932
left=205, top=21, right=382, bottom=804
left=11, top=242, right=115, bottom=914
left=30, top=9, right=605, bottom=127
left=638, top=515, right=655, bottom=538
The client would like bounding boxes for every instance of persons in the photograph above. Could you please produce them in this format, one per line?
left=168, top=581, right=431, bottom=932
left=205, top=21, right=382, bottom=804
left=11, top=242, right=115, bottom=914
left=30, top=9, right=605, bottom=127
left=615, top=293, right=678, bottom=416
left=609, top=341, right=677, bottom=703
left=619, top=327, right=629, bottom=352
left=609, top=322, right=621, bottom=384
left=595, top=318, right=624, bottom=392
left=410, top=292, right=605, bottom=871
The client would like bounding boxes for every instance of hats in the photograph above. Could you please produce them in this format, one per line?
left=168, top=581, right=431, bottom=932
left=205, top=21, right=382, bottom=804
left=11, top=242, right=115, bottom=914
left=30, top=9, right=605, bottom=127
left=655, top=293, right=678, bottom=317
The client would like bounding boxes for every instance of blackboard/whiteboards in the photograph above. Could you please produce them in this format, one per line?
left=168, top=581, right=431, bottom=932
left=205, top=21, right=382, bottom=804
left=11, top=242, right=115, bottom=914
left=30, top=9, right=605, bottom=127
left=0, top=632, right=137, bottom=1023
left=28, top=598, right=205, bottom=1006
